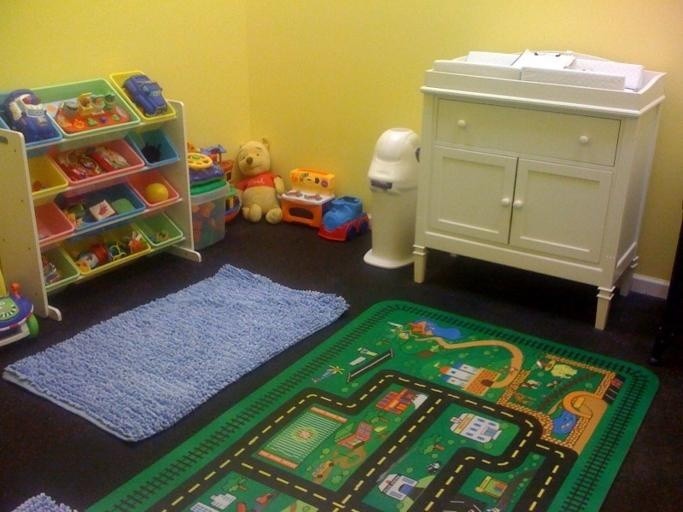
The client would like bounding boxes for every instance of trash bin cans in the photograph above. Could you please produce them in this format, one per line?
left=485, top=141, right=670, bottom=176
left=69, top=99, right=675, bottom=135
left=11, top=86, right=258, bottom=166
left=364, top=128, right=429, bottom=268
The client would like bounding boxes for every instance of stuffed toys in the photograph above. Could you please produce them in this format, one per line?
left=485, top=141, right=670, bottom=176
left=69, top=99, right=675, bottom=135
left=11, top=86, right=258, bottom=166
left=233, top=138, right=285, bottom=225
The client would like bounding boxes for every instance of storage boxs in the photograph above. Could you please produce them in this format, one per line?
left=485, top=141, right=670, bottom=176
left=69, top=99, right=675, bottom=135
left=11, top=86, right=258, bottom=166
left=60, top=223, right=153, bottom=286
left=191, top=178, right=225, bottom=250
left=56, top=184, right=146, bottom=240
left=0, top=88, right=62, bottom=149
left=26, top=155, right=69, bottom=208
left=26, top=201, right=76, bottom=253
left=30, top=78, right=142, bottom=153
left=30, top=247, right=81, bottom=294
left=131, top=211, right=185, bottom=257
left=48, top=138, right=147, bottom=200
left=124, top=128, right=182, bottom=170
left=122, top=170, right=180, bottom=220
left=107, top=70, right=178, bottom=135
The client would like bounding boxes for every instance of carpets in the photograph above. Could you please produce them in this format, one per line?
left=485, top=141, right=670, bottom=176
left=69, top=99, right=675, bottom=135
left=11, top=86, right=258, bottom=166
left=9, top=493, right=76, bottom=512
left=83, top=299, right=660, bottom=511
left=2, top=264, right=350, bottom=441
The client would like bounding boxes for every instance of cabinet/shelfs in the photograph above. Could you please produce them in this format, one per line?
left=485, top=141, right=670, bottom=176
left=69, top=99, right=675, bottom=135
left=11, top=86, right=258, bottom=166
left=0, top=99, right=202, bottom=322
left=411, top=48, right=668, bottom=333
